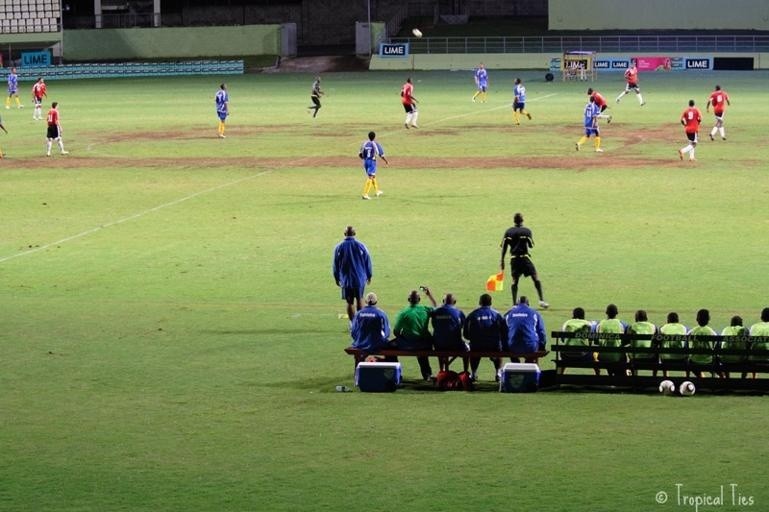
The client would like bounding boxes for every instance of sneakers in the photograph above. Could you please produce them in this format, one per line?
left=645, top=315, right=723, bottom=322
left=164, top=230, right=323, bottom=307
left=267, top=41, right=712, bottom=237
left=615, top=98, right=621, bottom=105
left=575, top=142, right=581, bottom=152
left=495, top=373, right=500, bottom=382
left=33, top=115, right=43, bottom=121
left=539, top=301, right=549, bottom=307
left=527, top=113, right=532, bottom=120
left=512, top=122, right=520, bottom=126
left=594, top=149, right=604, bottom=152
left=218, top=134, right=226, bottom=138
left=60, top=150, right=69, bottom=155
left=678, top=150, right=698, bottom=162
left=404, top=122, right=419, bottom=130
left=606, top=115, right=613, bottom=126
left=640, top=102, right=646, bottom=108
left=362, top=191, right=383, bottom=200
left=46, top=153, right=51, bottom=157
left=18, top=105, right=24, bottom=109
left=709, top=133, right=727, bottom=142
left=468, top=374, right=479, bottom=382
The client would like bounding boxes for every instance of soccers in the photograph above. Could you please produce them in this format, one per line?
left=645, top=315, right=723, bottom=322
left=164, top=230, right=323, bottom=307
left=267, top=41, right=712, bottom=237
left=659, top=380, right=675, bottom=395
left=413, top=29, right=423, bottom=38
left=681, top=381, right=696, bottom=396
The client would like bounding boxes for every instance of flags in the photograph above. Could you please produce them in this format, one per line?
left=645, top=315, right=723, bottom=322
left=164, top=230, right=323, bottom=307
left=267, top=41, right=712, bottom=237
left=485, top=271, right=505, bottom=291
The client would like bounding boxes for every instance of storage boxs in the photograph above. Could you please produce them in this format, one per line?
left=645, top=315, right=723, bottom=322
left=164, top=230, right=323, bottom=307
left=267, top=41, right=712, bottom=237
left=498, top=361, right=542, bottom=392
left=355, top=362, right=402, bottom=393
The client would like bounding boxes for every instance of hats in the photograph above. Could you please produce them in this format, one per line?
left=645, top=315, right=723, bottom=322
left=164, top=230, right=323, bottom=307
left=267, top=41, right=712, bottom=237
left=366, top=292, right=377, bottom=304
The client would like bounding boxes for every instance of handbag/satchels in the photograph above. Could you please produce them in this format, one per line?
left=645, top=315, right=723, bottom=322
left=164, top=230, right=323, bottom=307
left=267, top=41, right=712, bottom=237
left=432, top=369, right=478, bottom=392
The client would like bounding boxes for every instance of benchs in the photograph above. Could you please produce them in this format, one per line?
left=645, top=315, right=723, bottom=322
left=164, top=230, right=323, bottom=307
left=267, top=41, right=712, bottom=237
left=546, top=328, right=768, bottom=392
left=345, top=349, right=550, bottom=374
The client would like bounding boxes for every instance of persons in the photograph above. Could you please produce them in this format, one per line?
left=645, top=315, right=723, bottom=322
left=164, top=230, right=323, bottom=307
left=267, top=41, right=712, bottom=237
left=559, top=305, right=600, bottom=375
left=214, top=83, right=229, bottom=139
left=573, top=97, right=611, bottom=153
left=0, top=114, right=9, bottom=158
left=399, top=77, right=420, bottom=130
left=6, top=67, right=24, bottom=110
left=687, top=307, right=720, bottom=377
left=719, top=315, right=749, bottom=378
left=706, top=85, right=731, bottom=141
left=588, top=87, right=611, bottom=123
left=511, top=78, right=532, bottom=127
left=472, top=62, right=488, bottom=104
left=501, top=213, right=551, bottom=308
left=357, top=132, right=390, bottom=199
left=350, top=292, right=391, bottom=362
left=431, top=293, right=471, bottom=377
left=332, top=224, right=372, bottom=321
left=308, top=74, right=326, bottom=118
left=614, top=64, right=648, bottom=106
left=656, top=313, right=691, bottom=377
left=594, top=303, right=629, bottom=362
left=677, top=100, right=702, bottom=161
left=626, top=309, right=660, bottom=376
left=29, top=77, right=48, bottom=119
left=749, top=308, right=769, bottom=379
left=46, top=102, right=68, bottom=158
left=500, top=296, right=546, bottom=362
left=463, top=294, right=505, bottom=382
left=388, top=285, right=438, bottom=382
